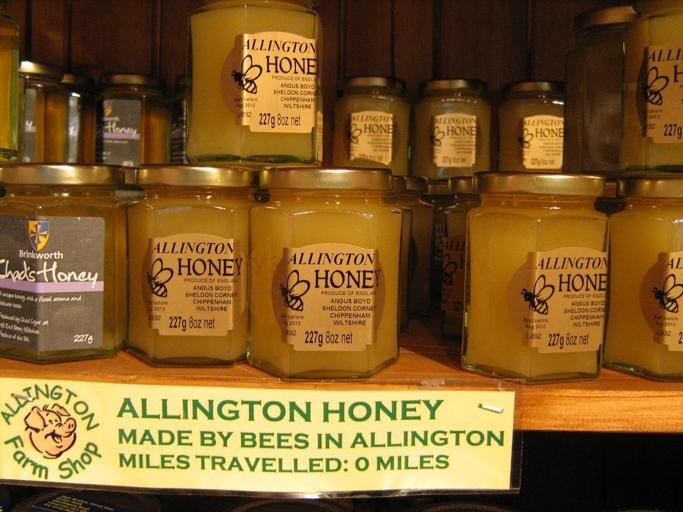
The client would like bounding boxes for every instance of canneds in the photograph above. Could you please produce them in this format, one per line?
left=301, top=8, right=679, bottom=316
left=0, top=0, right=683, bottom=382
